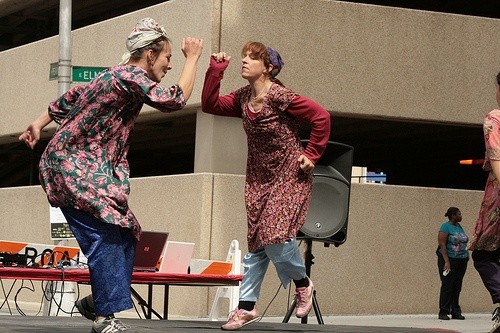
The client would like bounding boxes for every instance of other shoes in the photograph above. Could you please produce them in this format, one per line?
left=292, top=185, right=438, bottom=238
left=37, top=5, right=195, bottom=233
left=438, top=315, right=450, bottom=320
left=451, top=315, right=465, bottom=320
left=488, top=307, right=500, bottom=333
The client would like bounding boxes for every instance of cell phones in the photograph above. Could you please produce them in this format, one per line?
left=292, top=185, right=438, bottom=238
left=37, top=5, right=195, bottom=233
left=443, top=268, right=450, bottom=276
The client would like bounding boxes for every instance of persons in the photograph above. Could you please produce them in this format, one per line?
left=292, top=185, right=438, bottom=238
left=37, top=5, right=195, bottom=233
left=200, top=42, right=332, bottom=330
left=471, top=72, right=499, bottom=333
left=435, top=207, right=470, bottom=321
left=17, top=16, right=204, bottom=332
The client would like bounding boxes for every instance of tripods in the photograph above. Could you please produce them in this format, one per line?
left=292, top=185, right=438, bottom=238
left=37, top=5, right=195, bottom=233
left=281, top=238, right=325, bottom=326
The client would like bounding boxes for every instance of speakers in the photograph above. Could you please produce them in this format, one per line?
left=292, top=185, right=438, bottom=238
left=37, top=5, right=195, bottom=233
left=296, top=138, right=355, bottom=246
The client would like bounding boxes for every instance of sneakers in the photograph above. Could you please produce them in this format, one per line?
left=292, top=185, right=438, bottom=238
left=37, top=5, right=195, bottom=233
left=75, top=292, right=97, bottom=320
left=92, top=316, right=129, bottom=333
left=294, top=275, right=314, bottom=319
left=221, top=305, right=259, bottom=331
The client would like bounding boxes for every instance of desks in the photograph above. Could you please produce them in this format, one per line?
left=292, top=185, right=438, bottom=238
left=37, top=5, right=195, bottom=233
left=0, top=266, right=243, bottom=320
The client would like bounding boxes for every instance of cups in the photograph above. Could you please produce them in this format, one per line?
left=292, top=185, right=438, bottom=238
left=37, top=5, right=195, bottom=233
left=443, top=268, right=450, bottom=276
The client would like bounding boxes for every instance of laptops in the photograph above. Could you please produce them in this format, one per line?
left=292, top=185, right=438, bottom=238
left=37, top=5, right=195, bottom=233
left=156, top=242, right=195, bottom=274
left=133, top=231, right=169, bottom=272
